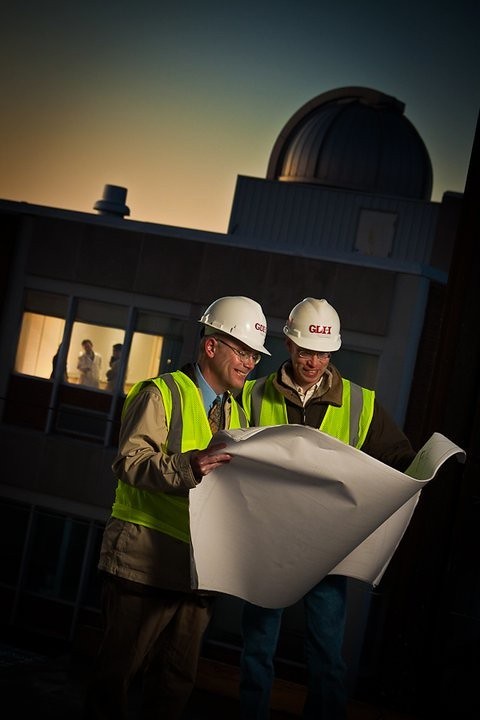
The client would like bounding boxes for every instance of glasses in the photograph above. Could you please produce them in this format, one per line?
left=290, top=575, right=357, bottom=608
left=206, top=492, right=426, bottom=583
left=291, top=342, right=331, bottom=361
left=217, top=339, right=261, bottom=365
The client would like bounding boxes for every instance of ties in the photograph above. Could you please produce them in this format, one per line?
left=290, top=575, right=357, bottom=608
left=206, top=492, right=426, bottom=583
left=209, top=397, right=224, bottom=434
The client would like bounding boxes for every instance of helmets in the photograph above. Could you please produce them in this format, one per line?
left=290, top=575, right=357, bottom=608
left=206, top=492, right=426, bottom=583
left=197, top=297, right=271, bottom=356
left=283, top=298, right=342, bottom=351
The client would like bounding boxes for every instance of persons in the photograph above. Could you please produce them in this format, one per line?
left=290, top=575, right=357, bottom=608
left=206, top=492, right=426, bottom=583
left=49, top=339, right=129, bottom=394
left=75, top=296, right=274, bottom=720
left=231, top=297, right=418, bottom=720
